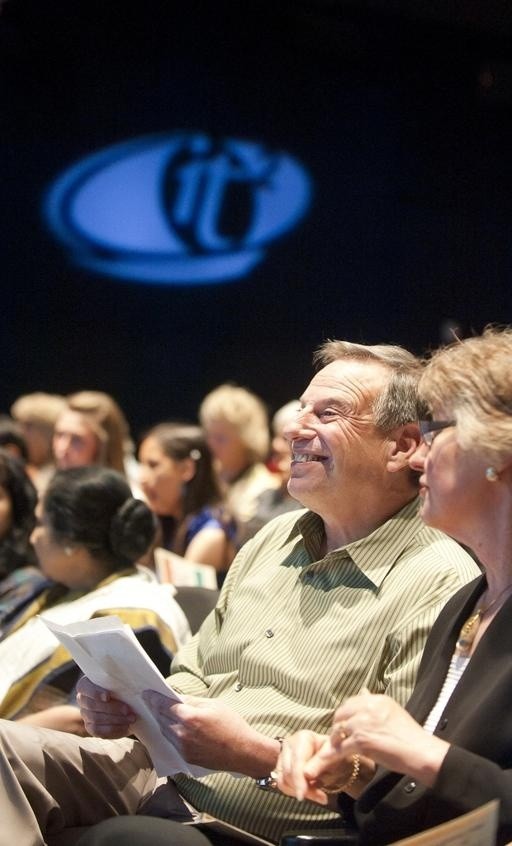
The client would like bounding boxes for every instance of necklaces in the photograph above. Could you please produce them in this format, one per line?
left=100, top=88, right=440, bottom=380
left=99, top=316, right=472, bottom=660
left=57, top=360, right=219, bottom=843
left=456, top=580, right=512, bottom=654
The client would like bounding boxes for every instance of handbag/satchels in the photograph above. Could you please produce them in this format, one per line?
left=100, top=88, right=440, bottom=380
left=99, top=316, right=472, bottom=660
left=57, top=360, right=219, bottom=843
left=315, top=729, right=363, bottom=796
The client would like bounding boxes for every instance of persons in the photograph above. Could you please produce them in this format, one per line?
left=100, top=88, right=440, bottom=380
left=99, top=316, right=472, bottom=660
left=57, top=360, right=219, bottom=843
left=0, top=338, right=486, bottom=845
left=275, top=324, right=512, bottom=846
left=0, top=387, right=305, bottom=739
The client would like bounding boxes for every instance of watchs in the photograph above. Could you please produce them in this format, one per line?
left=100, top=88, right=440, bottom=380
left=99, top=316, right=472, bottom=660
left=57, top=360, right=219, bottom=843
left=255, top=735, right=287, bottom=791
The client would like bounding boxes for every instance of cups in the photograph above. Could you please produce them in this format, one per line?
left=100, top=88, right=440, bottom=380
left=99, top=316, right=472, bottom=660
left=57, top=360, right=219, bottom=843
left=419, top=409, right=457, bottom=447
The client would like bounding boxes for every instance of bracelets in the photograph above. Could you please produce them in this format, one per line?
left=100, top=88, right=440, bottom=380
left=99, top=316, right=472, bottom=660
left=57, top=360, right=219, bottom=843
left=315, top=729, right=363, bottom=796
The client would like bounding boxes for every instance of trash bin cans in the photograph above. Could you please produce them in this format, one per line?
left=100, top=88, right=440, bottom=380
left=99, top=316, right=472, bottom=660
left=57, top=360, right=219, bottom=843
left=255, top=735, right=287, bottom=791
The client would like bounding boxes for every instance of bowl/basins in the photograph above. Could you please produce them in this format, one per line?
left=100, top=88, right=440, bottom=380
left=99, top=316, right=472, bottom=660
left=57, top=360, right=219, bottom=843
left=456, top=580, right=512, bottom=654
